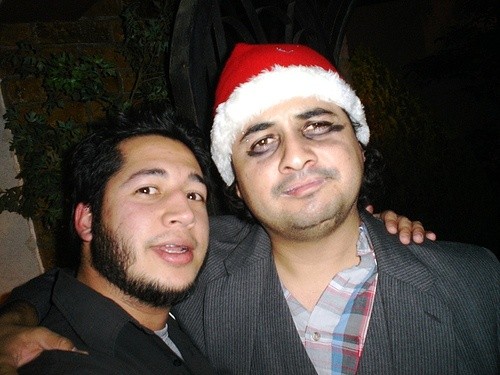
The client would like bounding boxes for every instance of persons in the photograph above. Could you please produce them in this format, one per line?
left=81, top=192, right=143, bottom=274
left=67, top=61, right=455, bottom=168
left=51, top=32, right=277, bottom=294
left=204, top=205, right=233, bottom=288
left=1, top=116, right=438, bottom=375
left=3, top=42, right=500, bottom=375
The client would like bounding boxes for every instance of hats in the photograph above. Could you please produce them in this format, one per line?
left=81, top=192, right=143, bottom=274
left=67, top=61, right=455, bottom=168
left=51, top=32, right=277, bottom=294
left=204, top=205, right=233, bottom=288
left=210, top=43, right=370, bottom=186
